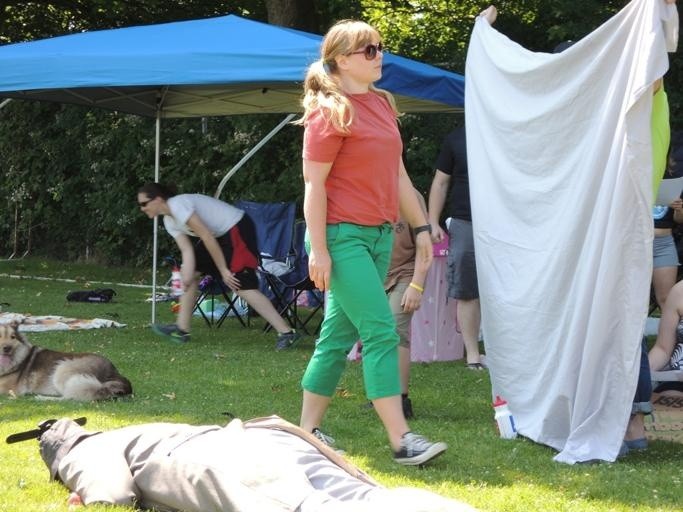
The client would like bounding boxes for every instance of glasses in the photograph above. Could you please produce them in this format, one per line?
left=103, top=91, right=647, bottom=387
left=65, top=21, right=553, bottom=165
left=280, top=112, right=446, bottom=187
left=137, top=199, right=153, bottom=206
left=348, top=42, right=384, bottom=60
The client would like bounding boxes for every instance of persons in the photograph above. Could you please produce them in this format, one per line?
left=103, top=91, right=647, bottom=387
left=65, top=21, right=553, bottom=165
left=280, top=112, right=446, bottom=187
left=427, top=123, right=483, bottom=371
left=651, top=169, right=681, bottom=322
left=644, top=279, right=681, bottom=412
left=37, top=413, right=479, bottom=512
left=477, top=0, right=672, bottom=456
left=136, top=182, right=300, bottom=355
left=297, top=17, right=449, bottom=465
left=375, top=185, right=427, bottom=422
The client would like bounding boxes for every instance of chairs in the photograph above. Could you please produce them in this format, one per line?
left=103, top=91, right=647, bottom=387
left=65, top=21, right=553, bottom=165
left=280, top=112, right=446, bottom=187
left=185, top=201, right=330, bottom=342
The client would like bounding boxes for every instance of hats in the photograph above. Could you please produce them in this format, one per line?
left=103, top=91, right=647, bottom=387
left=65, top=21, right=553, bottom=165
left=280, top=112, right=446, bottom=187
left=38, top=416, right=102, bottom=483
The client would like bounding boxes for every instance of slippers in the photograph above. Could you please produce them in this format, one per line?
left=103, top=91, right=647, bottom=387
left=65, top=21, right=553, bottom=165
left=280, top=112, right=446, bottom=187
left=465, top=363, right=483, bottom=371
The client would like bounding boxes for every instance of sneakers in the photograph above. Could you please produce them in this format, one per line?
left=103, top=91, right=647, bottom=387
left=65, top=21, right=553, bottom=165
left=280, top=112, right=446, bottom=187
left=619, top=438, right=648, bottom=458
left=312, top=428, right=346, bottom=453
left=370, top=399, right=414, bottom=419
left=391, top=432, right=448, bottom=467
left=277, top=329, right=301, bottom=350
left=152, top=323, right=190, bottom=343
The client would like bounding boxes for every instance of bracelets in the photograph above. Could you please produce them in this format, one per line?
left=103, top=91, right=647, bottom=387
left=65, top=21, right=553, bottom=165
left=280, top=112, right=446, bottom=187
left=413, top=226, right=433, bottom=233
left=407, top=279, right=423, bottom=294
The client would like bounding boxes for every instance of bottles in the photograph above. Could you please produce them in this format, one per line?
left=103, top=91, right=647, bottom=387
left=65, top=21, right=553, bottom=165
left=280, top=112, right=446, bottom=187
left=171, top=266, right=183, bottom=295
left=491, top=395, right=517, bottom=441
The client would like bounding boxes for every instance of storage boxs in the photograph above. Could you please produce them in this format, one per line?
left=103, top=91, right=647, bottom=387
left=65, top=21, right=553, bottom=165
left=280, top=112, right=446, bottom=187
left=641, top=391, right=683, bottom=443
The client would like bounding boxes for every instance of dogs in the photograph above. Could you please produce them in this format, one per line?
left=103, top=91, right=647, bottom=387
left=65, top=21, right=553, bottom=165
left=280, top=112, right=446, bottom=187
left=0, top=319, right=132, bottom=401
